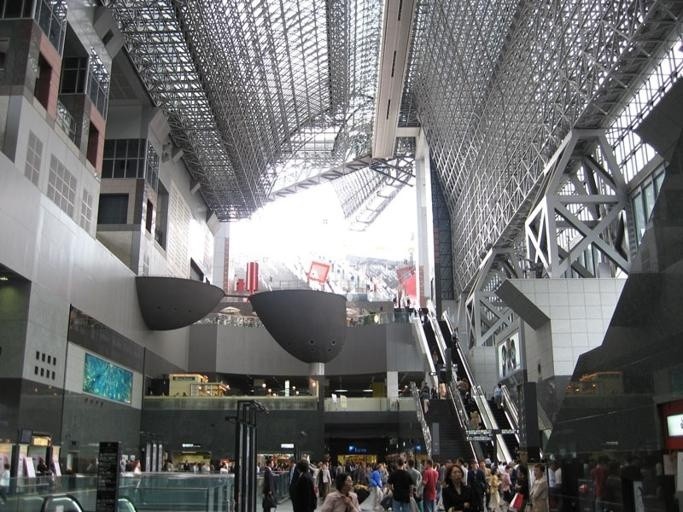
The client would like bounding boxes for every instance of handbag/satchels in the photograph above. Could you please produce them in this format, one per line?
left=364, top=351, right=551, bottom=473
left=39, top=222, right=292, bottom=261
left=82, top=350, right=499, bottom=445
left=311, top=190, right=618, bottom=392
left=508, top=491, right=524, bottom=512
left=262, top=491, right=278, bottom=509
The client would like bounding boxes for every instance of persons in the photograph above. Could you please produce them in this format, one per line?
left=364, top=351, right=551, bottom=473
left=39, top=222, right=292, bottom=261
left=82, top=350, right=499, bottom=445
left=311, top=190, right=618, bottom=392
left=0, top=462, right=10, bottom=506
left=120, top=457, right=143, bottom=472
left=162, top=458, right=211, bottom=473
left=219, top=458, right=235, bottom=474
left=35, top=456, right=47, bottom=476
left=402, top=328, right=518, bottom=453
left=260, top=452, right=676, bottom=511
